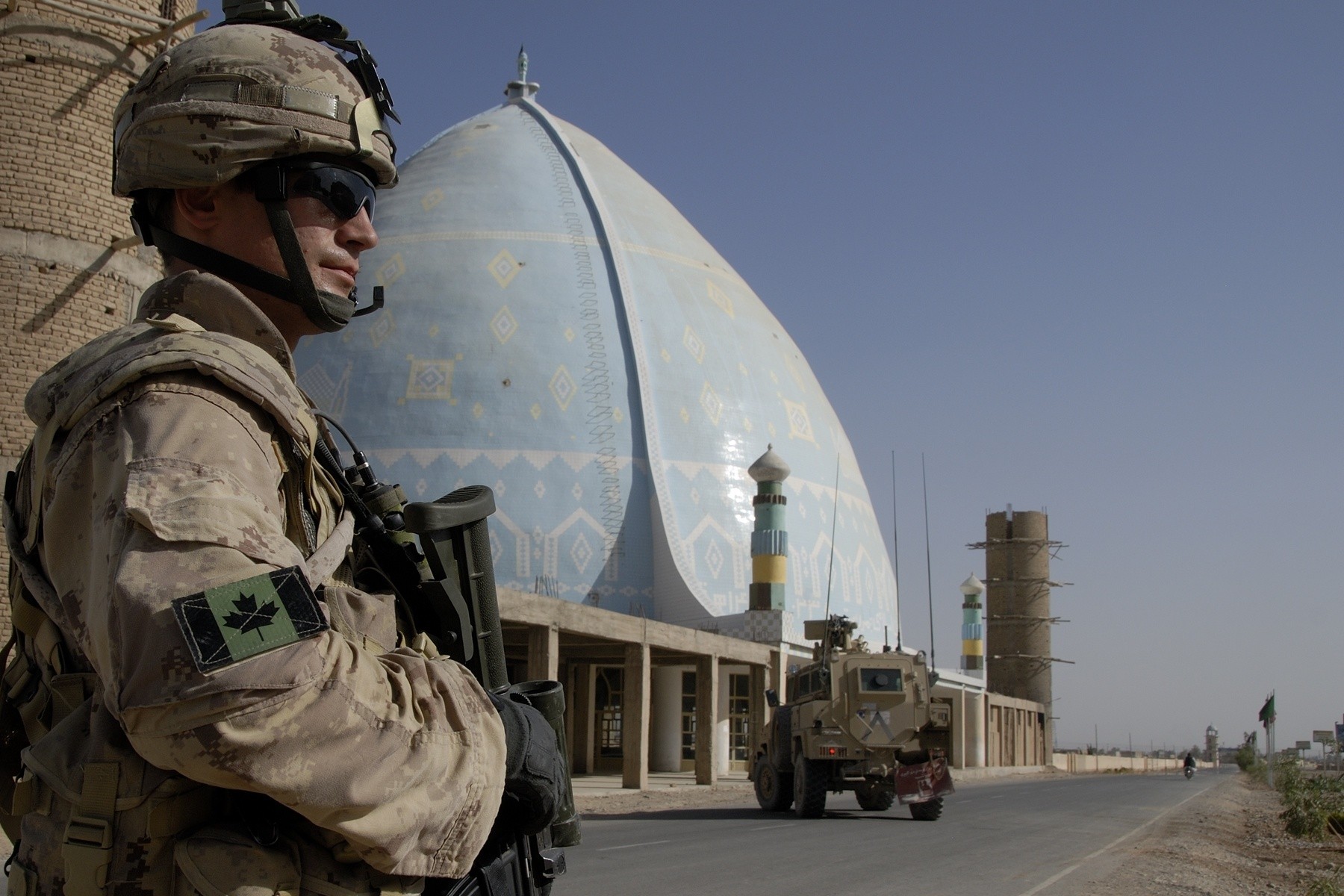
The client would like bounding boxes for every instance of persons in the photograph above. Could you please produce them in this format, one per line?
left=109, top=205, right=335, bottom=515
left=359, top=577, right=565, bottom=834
left=0, top=14, right=567, bottom=896
left=1184, top=753, right=1196, bottom=774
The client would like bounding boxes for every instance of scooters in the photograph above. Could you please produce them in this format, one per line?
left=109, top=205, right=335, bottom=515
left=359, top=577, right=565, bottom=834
left=1182, top=765, right=1195, bottom=780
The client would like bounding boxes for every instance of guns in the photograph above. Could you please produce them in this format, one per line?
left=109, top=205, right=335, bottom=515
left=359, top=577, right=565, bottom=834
left=402, top=482, right=584, bottom=896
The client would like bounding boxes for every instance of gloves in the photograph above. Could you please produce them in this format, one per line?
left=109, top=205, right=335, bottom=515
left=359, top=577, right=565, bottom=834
left=485, top=689, right=564, bottom=834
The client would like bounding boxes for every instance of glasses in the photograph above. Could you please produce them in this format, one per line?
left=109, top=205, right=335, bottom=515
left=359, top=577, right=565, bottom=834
left=242, top=160, right=378, bottom=224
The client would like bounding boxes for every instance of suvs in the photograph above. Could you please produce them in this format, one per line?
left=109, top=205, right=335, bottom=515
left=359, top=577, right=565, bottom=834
left=747, top=449, right=957, bottom=821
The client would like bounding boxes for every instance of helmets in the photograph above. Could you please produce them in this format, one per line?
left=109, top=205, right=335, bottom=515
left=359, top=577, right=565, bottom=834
left=111, top=24, right=400, bottom=197
